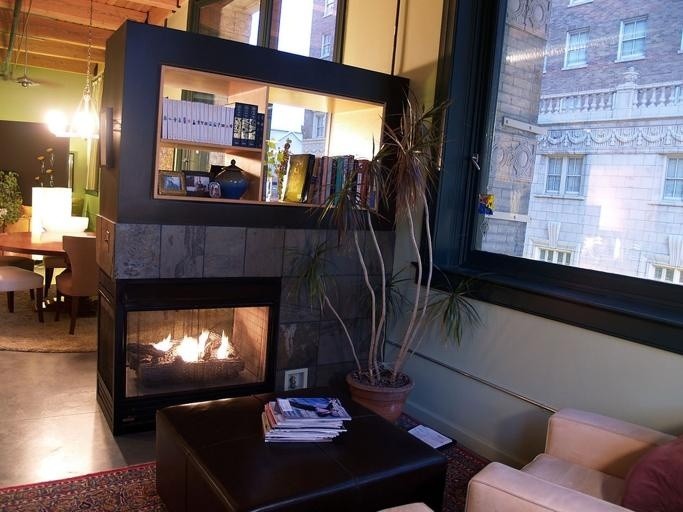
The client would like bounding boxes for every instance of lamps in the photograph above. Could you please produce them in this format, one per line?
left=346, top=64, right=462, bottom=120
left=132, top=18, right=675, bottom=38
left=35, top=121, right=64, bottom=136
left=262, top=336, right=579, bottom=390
left=36, top=1, right=102, bottom=142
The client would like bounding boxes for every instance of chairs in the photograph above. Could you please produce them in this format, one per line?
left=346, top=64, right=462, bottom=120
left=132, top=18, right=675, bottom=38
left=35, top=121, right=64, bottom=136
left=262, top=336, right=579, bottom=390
left=0, top=214, right=97, bottom=335
left=459, top=401, right=682, bottom=511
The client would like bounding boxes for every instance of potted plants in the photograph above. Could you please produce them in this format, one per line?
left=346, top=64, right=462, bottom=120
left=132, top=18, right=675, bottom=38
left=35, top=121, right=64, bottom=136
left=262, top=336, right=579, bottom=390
left=294, top=95, right=480, bottom=419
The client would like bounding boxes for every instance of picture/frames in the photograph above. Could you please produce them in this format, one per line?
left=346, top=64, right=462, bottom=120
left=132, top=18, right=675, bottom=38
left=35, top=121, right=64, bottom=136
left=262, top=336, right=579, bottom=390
left=157, top=169, right=186, bottom=196
left=94, top=106, right=114, bottom=169
left=84, top=138, right=100, bottom=198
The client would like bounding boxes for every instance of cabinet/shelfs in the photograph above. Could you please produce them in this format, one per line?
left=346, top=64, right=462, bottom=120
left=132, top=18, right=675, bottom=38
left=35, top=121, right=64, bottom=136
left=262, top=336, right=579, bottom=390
left=147, top=64, right=386, bottom=214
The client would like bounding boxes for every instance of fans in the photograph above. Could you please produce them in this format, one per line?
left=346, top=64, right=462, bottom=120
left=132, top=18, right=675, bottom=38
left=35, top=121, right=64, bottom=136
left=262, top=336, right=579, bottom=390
left=0, top=14, right=68, bottom=89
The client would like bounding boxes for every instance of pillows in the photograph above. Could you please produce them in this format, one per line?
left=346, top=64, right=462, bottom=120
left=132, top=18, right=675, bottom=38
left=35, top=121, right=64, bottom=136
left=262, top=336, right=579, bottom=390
left=620, top=434, right=682, bottom=511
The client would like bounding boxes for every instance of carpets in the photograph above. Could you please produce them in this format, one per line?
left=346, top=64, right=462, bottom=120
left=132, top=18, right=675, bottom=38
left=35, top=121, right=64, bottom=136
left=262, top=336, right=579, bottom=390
left=0, top=413, right=489, bottom=512
left=0, top=286, right=99, bottom=355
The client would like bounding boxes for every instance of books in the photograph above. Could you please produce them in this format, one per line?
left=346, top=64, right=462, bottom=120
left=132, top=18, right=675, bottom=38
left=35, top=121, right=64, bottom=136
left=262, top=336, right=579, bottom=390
left=160, top=98, right=263, bottom=150
left=282, top=152, right=377, bottom=208
left=259, top=395, right=353, bottom=446
